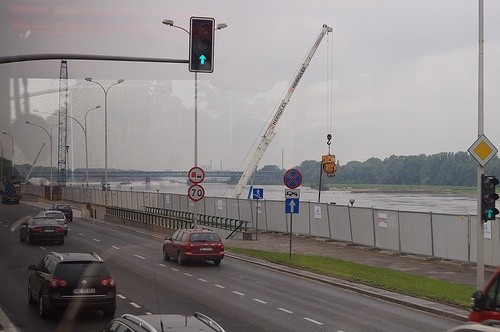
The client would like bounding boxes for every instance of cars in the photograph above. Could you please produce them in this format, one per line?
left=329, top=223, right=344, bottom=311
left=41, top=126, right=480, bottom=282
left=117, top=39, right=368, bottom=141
left=38, top=210, right=68, bottom=235
left=20, top=216, right=64, bottom=245
left=51, top=204, right=73, bottom=222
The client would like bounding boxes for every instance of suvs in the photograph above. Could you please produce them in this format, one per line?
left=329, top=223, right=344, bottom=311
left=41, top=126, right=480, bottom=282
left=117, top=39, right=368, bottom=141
left=104, top=300, right=226, bottom=332
left=162, top=227, right=225, bottom=267
left=28, top=250, right=116, bottom=323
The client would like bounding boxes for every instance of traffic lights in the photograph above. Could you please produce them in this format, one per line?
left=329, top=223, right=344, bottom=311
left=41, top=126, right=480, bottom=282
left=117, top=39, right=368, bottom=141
left=187, top=16, right=216, bottom=74
left=480, top=173, right=500, bottom=222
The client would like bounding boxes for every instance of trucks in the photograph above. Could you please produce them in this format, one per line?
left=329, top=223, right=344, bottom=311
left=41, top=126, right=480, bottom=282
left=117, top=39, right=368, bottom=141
left=468, top=266, right=500, bottom=326
left=0, top=179, right=21, bottom=204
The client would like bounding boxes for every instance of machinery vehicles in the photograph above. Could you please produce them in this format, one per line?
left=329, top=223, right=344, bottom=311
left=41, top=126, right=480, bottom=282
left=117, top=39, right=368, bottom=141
left=25, top=141, right=46, bottom=182
left=224, top=23, right=338, bottom=198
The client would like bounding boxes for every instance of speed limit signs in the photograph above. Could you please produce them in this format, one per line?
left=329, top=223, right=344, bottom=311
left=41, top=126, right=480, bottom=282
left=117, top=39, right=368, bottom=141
left=187, top=184, right=205, bottom=201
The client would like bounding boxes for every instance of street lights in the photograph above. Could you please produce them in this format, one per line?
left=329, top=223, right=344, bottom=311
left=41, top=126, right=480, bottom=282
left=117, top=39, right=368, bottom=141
left=2, top=131, right=15, bottom=179
left=25, top=121, right=59, bottom=200
left=32, top=105, right=102, bottom=186
left=85, top=76, right=125, bottom=191
